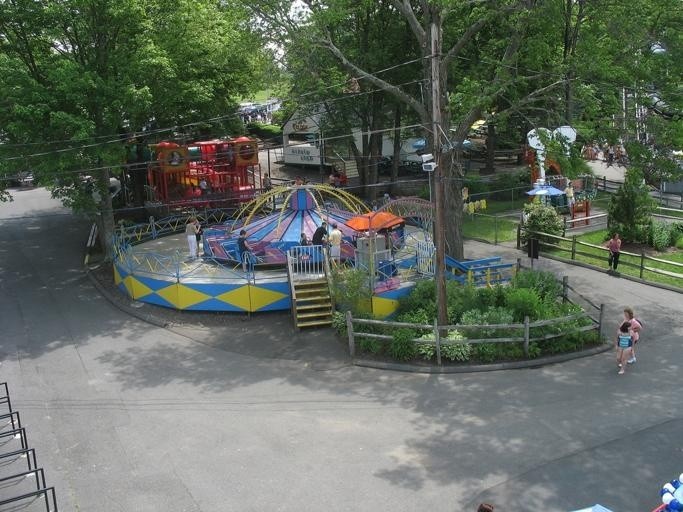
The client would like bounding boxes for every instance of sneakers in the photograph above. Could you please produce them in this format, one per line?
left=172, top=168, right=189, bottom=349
left=617, top=357, right=636, bottom=375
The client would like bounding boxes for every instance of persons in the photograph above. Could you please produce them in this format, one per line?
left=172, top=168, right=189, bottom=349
left=618, top=308, right=643, bottom=364
left=563, top=182, right=576, bottom=219
left=312, top=222, right=331, bottom=247
left=581, top=138, right=627, bottom=165
left=325, top=223, right=341, bottom=269
left=474, top=499, right=498, bottom=512
left=295, top=176, right=303, bottom=186
left=240, top=108, right=273, bottom=126
left=328, top=171, right=347, bottom=187
left=194, top=219, right=203, bottom=257
left=199, top=177, right=208, bottom=193
left=606, top=232, right=622, bottom=272
left=615, top=321, right=635, bottom=375
left=298, top=232, right=311, bottom=246
left=263, top=173, right=271, bottom=192
left=237, top=229, right=253, bottom=273
left=184, top=216, right=201, bottom=259
